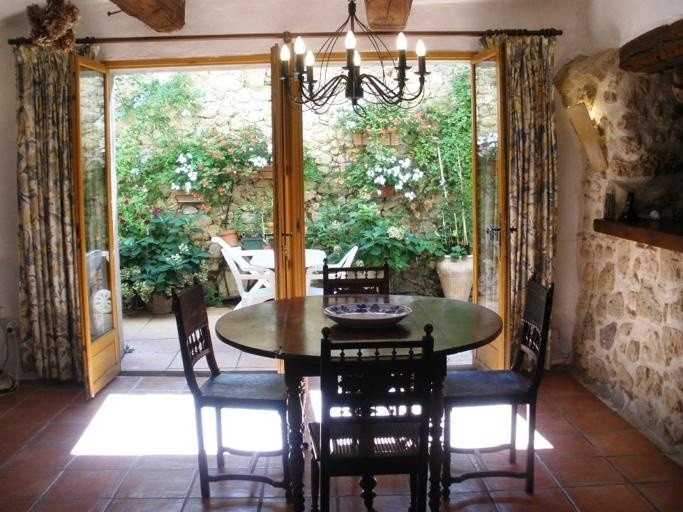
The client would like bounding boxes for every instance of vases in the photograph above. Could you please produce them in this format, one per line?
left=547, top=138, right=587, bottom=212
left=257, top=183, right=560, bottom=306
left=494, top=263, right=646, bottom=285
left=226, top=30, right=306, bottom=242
left=137, top=289, right=171, bottom=315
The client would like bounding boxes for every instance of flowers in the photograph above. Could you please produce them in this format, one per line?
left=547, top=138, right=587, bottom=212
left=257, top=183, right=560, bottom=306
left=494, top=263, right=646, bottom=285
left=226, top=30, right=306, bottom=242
left=310, top=143, right=431, bottom=268
left=119, top=130, right=272, bottom=300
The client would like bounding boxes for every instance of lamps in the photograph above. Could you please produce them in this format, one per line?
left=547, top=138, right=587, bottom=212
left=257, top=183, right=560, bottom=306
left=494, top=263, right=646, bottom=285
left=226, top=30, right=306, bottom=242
left=277, top=0, right=432, bottom=119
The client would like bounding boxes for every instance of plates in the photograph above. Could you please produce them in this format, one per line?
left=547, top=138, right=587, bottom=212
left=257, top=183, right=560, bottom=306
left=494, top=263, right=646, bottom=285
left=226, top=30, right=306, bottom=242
left=324, top=302, right=413, bottom=327
